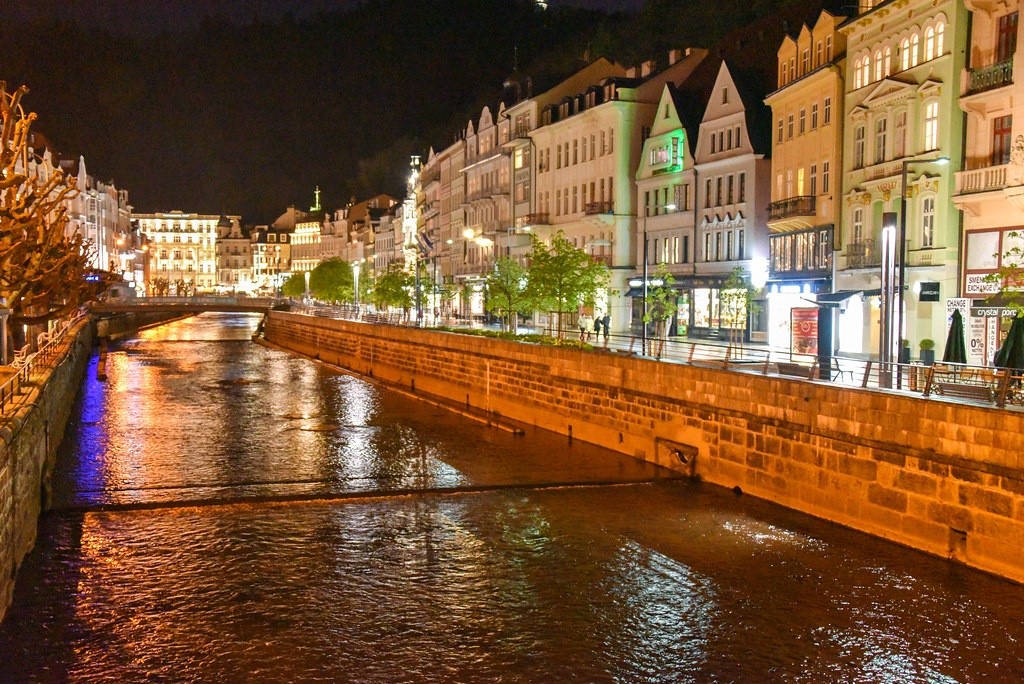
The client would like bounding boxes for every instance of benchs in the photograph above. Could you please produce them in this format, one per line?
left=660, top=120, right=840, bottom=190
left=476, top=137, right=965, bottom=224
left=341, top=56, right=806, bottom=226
left=934, top=381, right=993, bottom=404
left=8, top=343, right=31, bottom=368
left=817, top=357, right=854, bottom=383
left=37, top=320, right=59, bottom=350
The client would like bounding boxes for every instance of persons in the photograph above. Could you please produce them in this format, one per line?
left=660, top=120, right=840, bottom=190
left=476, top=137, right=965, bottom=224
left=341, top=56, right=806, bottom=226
left=575, top=311, right=611, bottom=342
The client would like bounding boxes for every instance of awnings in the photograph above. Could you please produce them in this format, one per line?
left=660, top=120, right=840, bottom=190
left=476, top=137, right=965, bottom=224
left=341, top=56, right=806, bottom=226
left=621, top=287, right=675, bottom=299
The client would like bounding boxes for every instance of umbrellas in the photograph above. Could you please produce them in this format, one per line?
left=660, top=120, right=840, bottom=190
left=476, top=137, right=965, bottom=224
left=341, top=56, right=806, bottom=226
left=941, top=308, right=968, bottom=383
left=994, top=310, right=1024, bottom=389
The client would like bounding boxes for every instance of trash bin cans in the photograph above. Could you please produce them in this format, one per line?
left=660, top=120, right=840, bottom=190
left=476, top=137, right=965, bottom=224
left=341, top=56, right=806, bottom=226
left=908, top=364, right=917, bottom=392
left=917, top=366, right=932, bottom=391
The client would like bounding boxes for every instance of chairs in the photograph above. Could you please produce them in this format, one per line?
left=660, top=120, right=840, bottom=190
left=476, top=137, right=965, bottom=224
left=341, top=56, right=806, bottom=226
left=925, top=364, right=1024, bottom=405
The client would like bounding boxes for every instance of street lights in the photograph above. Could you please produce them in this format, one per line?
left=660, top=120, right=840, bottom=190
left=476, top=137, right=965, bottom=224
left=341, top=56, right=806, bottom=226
left=897, top=156, right=950, bottom=390
left=434, top=239, right=453, bottom=326
left=642, top=204, right=677, bottom=355
left=506, top=226, right=531, bottom=283
